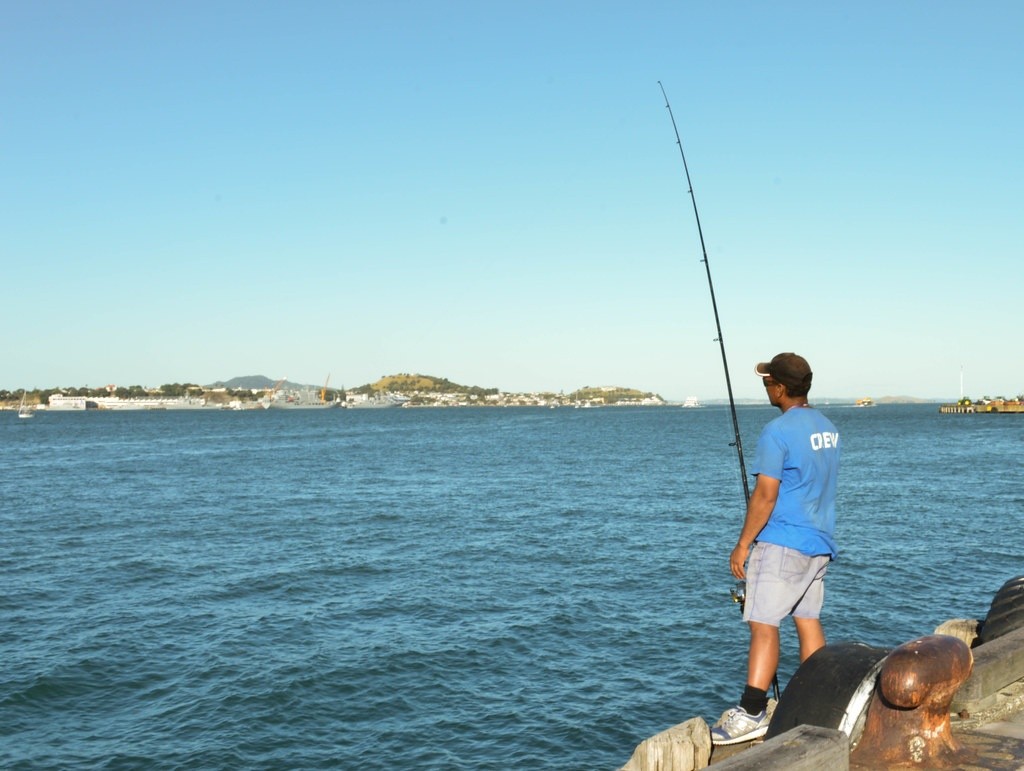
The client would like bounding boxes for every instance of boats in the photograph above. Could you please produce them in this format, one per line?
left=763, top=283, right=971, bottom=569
left=270, top=371, right=340, bottom=410
left=345, top=396, right=405, bottom=409
left=18, top=387, right=36, bottom=418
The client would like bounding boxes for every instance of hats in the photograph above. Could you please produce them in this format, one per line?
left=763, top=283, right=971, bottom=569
left=756, top=352, right=813, bottom=386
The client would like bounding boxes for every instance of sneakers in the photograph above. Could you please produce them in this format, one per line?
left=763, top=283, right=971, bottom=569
left=710, top=707, right=772, bottom=745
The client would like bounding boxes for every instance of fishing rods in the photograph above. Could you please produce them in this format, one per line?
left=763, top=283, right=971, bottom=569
left=657, top=78, right=782, bottom=705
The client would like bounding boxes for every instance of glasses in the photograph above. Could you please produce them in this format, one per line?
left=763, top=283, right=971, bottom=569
left=762, top=379, right=780, bottom=387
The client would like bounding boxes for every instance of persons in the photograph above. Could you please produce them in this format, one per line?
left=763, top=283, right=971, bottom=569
left=711, top=352, right=840, bottom=745
